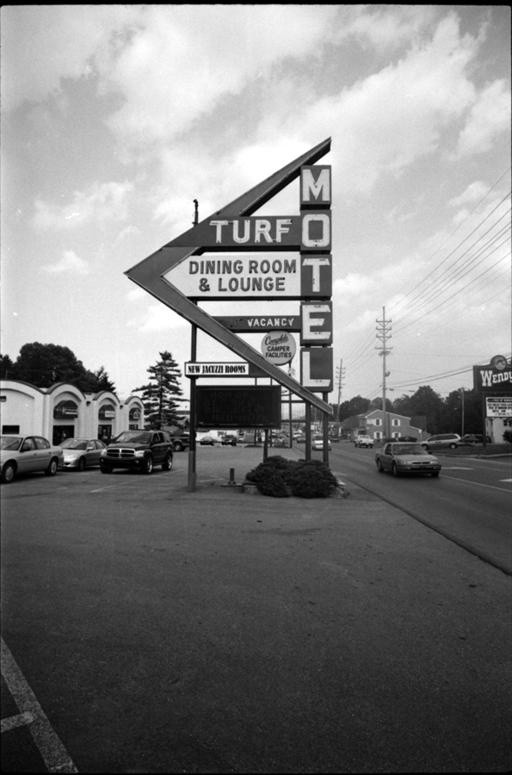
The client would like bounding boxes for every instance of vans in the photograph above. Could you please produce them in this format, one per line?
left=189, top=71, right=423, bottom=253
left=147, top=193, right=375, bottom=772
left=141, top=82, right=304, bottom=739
left=420, top=433, right=460, bottom=451
left=463, top=433, right=482, bottom=442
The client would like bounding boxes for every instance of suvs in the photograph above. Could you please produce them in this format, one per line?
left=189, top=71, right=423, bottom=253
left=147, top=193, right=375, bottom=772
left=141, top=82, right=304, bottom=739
left=98, top=429, right=175, bottom=474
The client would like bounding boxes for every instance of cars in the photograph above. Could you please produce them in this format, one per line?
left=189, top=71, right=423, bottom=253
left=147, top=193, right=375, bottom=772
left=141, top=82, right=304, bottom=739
left=390, top=436, right=417, bottom=442
left=56, top=438, right=109, bottom=472
left=1, top=434, right=65, bottom=483
left=222, top=435, right=236, bottom=447
left=376, top=441, right=441, bottom=480
left=200, top=436, right=216, bottom=446
left=273, top=428, right=340, bottom=451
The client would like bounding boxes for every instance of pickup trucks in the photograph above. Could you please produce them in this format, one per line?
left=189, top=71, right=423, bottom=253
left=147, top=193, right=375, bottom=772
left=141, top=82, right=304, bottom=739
left=171, top=434, right=191, bottom=452
left=353, top=435, right=374, bottom=448
